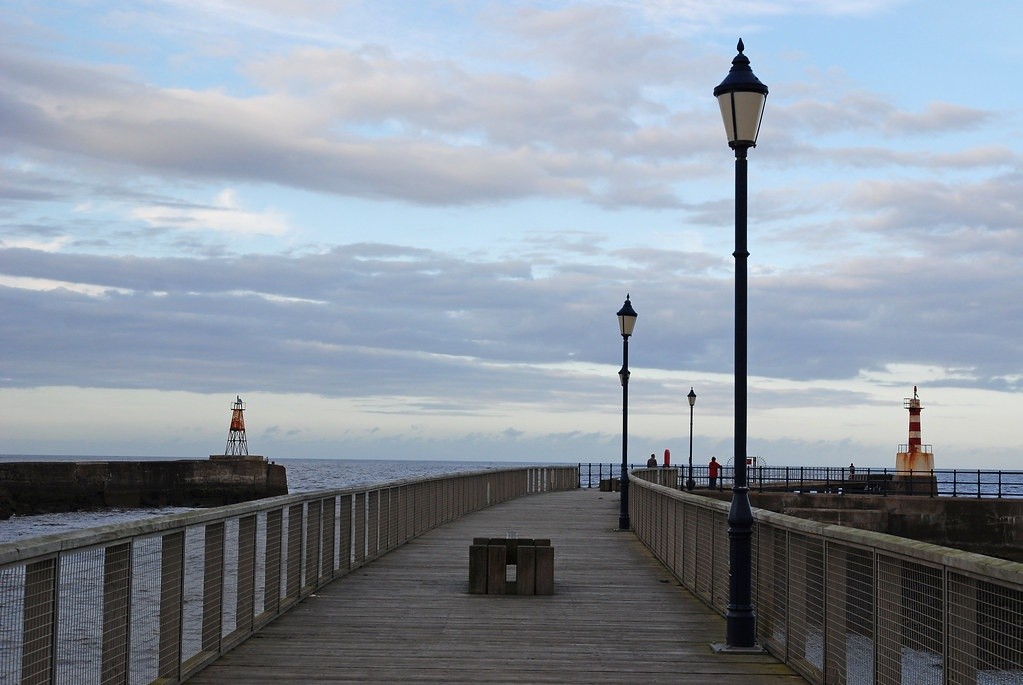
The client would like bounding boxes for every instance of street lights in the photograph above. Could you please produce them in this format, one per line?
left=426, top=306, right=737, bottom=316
left=687, top=387, right=697, bottom=489
left=713, top=37, right=770, bottom=650
left=614, top=292, right=638, bottom=531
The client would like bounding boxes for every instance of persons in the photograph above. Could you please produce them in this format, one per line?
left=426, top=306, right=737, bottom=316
left=647, top=454, right=657, bottom=468
left=709, top=456, right=721, bottom=489
left=849, top=463, right=855, bottom=474
